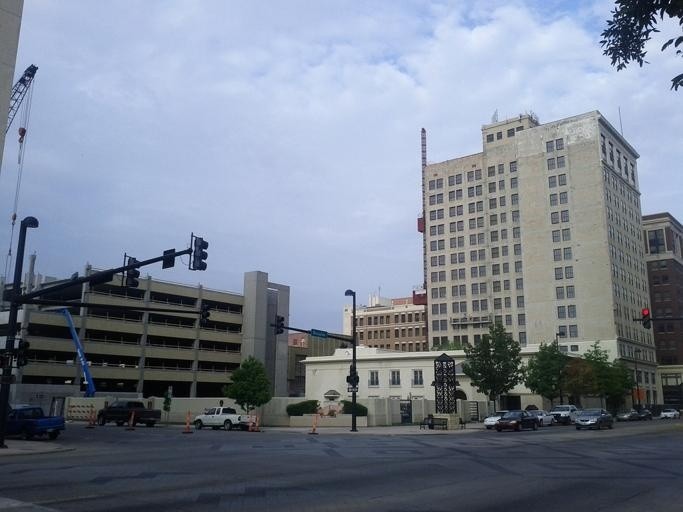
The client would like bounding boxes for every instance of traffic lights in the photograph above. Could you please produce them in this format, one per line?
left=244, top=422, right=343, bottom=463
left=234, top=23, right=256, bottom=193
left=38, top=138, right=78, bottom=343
left=17, top=341, right=30, bottom=367
left=201, top=305, right=209, bottom=326
left=641, top=309, right=651, bottom=329
left=193, top=237, right=208, bottom=269
left=276, top=315, right=284, bottom=334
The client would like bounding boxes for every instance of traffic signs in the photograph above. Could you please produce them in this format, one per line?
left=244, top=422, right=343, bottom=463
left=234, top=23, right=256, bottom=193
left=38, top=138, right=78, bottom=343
left=126, top=257, right=140, bottom=288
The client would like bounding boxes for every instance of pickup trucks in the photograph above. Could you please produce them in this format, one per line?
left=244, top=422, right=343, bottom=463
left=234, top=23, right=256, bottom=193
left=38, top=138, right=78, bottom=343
left=195, top=407, right=257, bottom=431
left=97, top=400, right=161, bottom=426
left=6, top=407, right=65, bottom=440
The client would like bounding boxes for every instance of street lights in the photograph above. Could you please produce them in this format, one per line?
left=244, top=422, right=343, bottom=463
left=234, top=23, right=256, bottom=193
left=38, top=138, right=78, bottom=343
left=1, top=216, right=39, bottom=450
left=634, top=349, right=641, bottom=408
left=345, top=289, right=359, bottom=431
left=556, top=332, right=565, bottom=405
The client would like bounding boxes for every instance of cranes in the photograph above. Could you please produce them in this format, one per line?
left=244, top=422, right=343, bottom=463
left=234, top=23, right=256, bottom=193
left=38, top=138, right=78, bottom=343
left=6, top=65, right=38, bottom=278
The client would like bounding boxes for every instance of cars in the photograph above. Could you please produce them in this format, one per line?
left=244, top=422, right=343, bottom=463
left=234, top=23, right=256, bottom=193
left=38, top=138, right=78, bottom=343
left=484, top=405, right=683, bottom=430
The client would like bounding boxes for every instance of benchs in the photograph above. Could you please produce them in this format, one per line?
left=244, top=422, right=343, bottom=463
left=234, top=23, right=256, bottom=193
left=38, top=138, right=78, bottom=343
left=419, top=417, right=448, bottom=430
left=459, top=417, right=466, bottom=430
